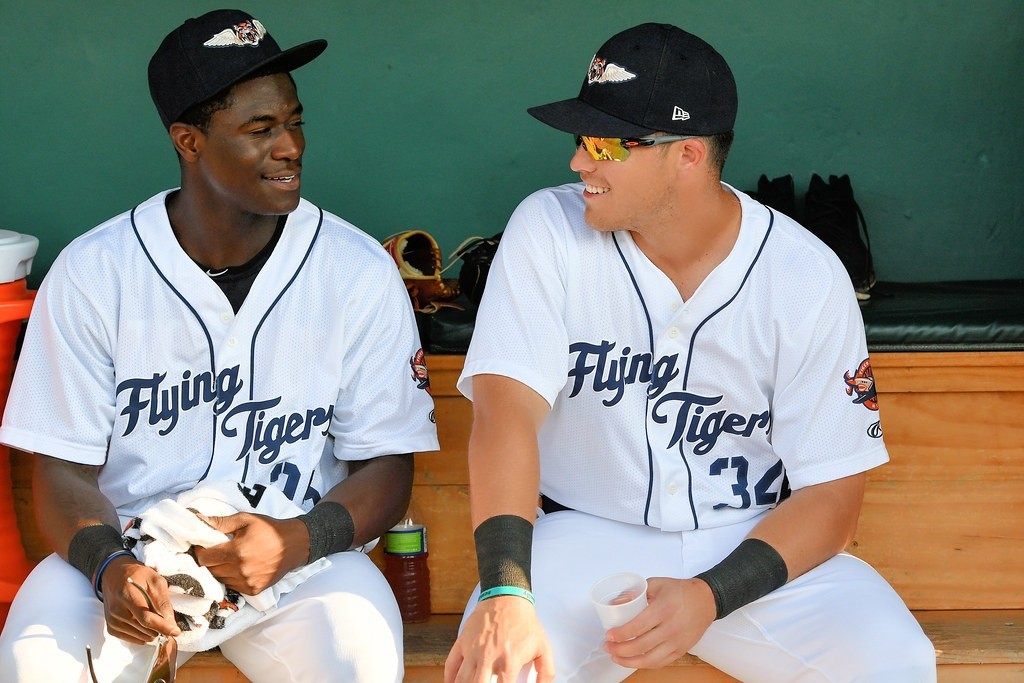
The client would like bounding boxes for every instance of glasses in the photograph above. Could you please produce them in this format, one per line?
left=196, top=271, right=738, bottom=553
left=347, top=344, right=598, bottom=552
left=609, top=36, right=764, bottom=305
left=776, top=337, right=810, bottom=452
left=574, top=131, right=701, bottom=162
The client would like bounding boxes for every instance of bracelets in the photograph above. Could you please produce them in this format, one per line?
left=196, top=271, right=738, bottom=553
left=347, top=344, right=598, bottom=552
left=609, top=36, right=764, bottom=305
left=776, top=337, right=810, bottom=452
left=91, top=550, right=137, bottom=604
left=478, top=585, right=536, bottom=606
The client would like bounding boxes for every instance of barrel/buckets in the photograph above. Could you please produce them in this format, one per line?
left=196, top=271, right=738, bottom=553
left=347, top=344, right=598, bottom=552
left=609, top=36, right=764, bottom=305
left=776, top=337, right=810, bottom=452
left=0, top=229, right=39, bottom=634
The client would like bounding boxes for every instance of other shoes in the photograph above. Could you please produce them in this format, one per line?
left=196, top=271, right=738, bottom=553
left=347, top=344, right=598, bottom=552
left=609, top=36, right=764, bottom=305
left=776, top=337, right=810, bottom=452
left=412, top=230, right=504, bottom=354
left=747, top=171, right=875, bottom=299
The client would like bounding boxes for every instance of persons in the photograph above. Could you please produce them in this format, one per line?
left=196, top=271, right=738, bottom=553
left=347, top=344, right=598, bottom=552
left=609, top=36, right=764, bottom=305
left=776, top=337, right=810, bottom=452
left=442, top=22, right=940, bottom=683
left=1, top=8, right=432, bottom=683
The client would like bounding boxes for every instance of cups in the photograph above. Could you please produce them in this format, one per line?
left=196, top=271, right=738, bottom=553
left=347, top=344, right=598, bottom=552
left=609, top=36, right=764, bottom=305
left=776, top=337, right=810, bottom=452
left=591, top=571, right=648, bottom=646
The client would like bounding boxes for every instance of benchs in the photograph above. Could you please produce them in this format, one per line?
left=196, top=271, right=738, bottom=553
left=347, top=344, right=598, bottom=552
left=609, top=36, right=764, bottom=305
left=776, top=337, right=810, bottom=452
left=171, top=353, right=1024, bottom=683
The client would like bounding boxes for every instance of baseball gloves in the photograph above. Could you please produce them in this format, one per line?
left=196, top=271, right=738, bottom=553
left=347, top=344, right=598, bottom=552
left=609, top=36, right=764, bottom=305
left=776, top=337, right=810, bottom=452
left=385, top=228, right=464, bottom=322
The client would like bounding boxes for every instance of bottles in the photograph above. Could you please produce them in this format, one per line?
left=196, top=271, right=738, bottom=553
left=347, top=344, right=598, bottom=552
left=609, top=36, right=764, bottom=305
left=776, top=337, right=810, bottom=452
left=381, top=496, right=431, bottom=624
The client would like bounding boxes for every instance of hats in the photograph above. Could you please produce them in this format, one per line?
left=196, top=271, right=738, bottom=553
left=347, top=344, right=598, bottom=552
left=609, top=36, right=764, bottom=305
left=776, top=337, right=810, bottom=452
left=527, top=22, right=739, bottom=138
left=147, top=9, right=328, bottom=132
